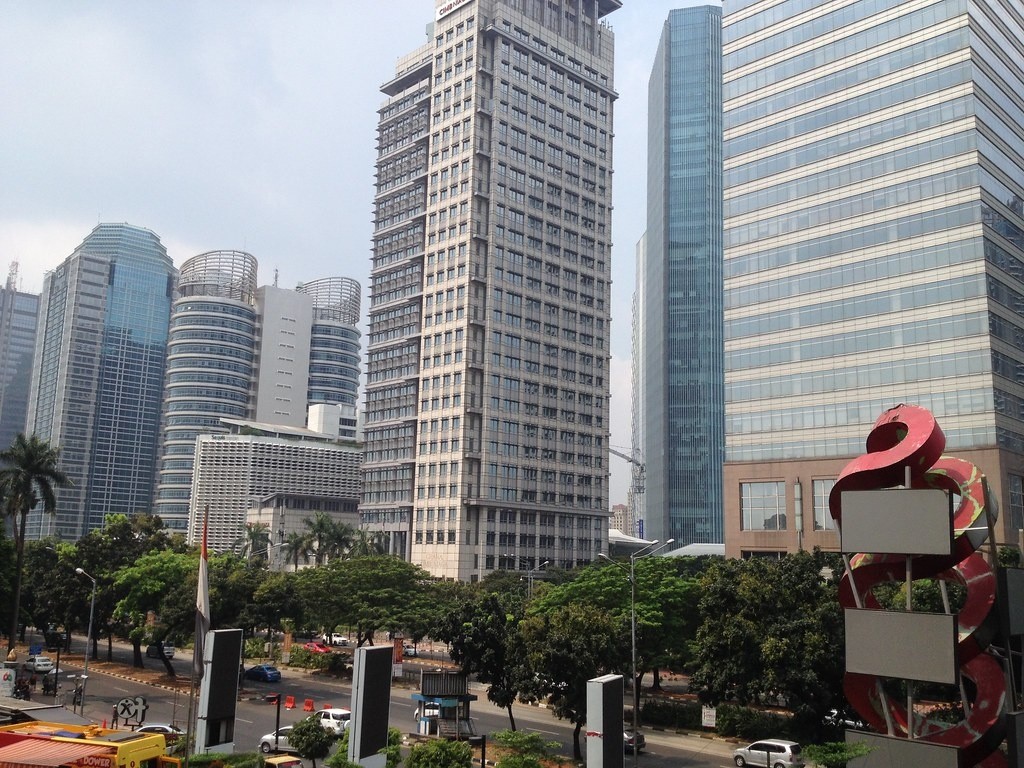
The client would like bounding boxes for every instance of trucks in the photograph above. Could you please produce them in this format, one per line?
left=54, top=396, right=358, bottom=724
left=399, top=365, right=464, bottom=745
left=0, top=720, right=182, bottom=768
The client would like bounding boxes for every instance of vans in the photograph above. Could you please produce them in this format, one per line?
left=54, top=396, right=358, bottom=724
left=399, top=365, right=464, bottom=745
left=146, top=640, right=175, bottom=659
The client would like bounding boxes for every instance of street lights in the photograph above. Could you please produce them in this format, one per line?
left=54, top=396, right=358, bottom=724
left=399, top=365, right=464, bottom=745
left=74, top=568, right=97, bottom=717
left=504, top=552, right=551, bottom=600
left=595, top=537, right=677, bottom=767
left=247, top=542, right=290, bottom=567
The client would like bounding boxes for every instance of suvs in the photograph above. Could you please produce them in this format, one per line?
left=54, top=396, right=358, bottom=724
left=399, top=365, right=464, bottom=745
left=733, top=739, right=806, bottom=768
left=306, top=708, right=352, bottom=741
left=46, top=631, right=72, bottom=646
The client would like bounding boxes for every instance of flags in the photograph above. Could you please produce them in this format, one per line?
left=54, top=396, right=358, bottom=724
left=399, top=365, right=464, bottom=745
left=193, top=509, right=211, bottom=678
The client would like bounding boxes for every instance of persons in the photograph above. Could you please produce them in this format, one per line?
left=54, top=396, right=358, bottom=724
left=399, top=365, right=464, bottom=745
left=14, top=674, right=82, bottom=705
left=111, top=704, right=118, bottom=729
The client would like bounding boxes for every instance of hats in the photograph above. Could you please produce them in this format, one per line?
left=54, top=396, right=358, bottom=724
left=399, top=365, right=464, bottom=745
left=113, top=704, right=117, bottom=708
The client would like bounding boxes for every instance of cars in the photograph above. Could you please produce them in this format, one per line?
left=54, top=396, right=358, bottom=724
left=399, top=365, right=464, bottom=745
left=824, top=708, right=864, bottom=729
left=583, top=726, right=647, bottom=754
left=414, top=702, right=462, bottom=724
left=133, top=723, right=185, bottom=743
left=403, top=644, right=418, bottom=657
left=244, top=664, right=281, bottom=682
left=259, top=724, right=327, bottom=757
left=22, top=657, right=55, bottom=674
left=304, top=642, right=331, bottom=655
left=322, top=632, right=349, bottom=646
